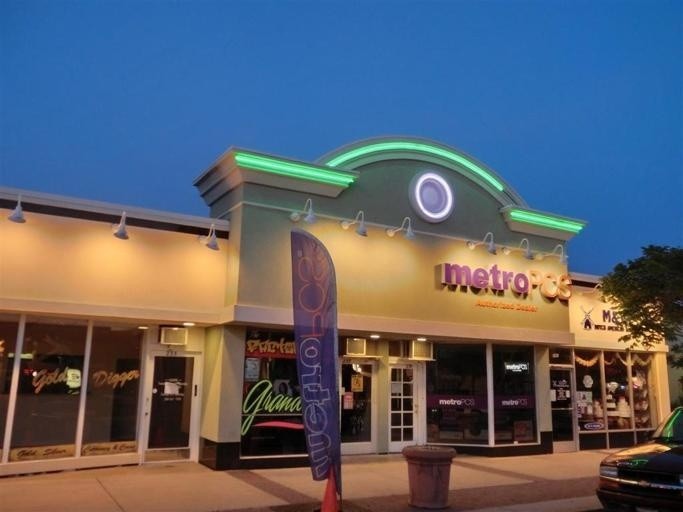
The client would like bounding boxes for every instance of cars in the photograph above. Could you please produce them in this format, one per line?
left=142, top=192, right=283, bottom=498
left=593, top=403, right=683, bottom=512
